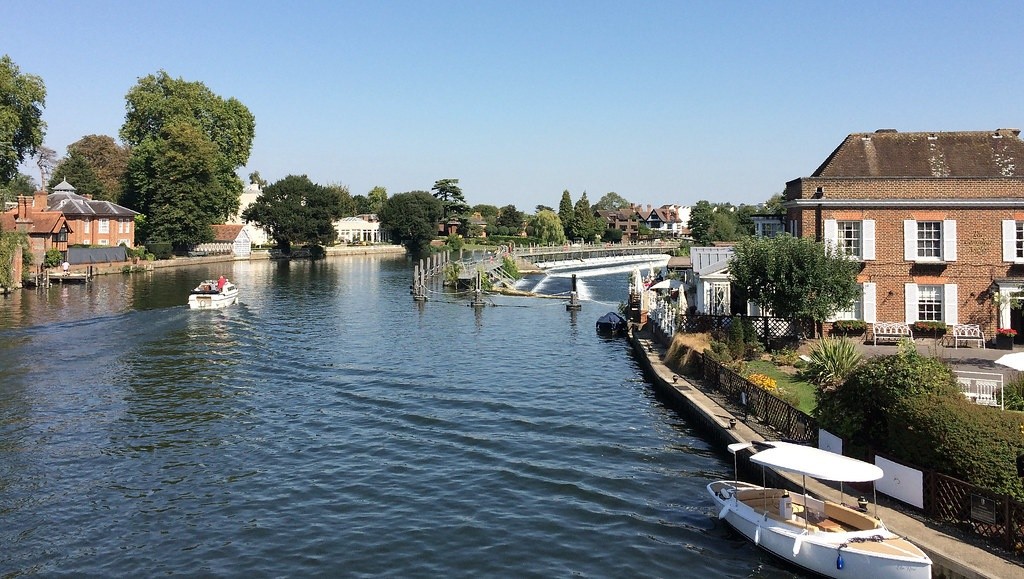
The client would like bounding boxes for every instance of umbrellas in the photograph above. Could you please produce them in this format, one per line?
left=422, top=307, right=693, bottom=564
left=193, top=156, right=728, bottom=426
left=650, top=279, right=689, bottom=294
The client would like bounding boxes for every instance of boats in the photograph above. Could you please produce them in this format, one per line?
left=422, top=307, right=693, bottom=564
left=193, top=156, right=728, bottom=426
left=596, top=313, right=624, bottom=338
left=188, top=278, right=239, bottom=310
left=707, top=441, right=933, bottom=579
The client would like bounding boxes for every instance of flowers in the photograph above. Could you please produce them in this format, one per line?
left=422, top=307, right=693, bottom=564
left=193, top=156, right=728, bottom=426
left=997, top=327, right=1017, bottom=337
left=830, top=319, right=868, bottom=331
left=913, top=320, right=948, bottom=330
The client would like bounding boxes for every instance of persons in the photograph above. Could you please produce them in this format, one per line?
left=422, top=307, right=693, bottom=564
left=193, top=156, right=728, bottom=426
left=218, top=276, right=225, bottom=293
left=644, top=275, right=664, bottom=289
left=62, top=260, right=70, bottom=274
left=489, top=243, right=512, bottom=263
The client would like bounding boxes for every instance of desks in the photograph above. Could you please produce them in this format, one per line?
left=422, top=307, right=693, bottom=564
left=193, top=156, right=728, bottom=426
left=942, top=335, right=955, bottom=347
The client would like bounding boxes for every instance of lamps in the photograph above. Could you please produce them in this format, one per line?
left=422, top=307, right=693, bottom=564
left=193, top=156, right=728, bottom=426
left=889, top=290, right=893, bottom=296
left=988, top=283, right=998, bottom=298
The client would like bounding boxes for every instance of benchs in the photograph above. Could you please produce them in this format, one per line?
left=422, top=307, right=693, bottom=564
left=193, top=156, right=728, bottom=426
left=872, top=321, right=913, bottom=348
left=953, top=324, right=986, bottom=350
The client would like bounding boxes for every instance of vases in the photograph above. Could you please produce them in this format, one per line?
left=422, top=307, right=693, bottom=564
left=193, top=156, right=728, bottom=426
left=995, top=335, right=1013, bottom=350
left=912, top=325, right=947, bottom=339
left=833, top=328, right=867, bottom=336
